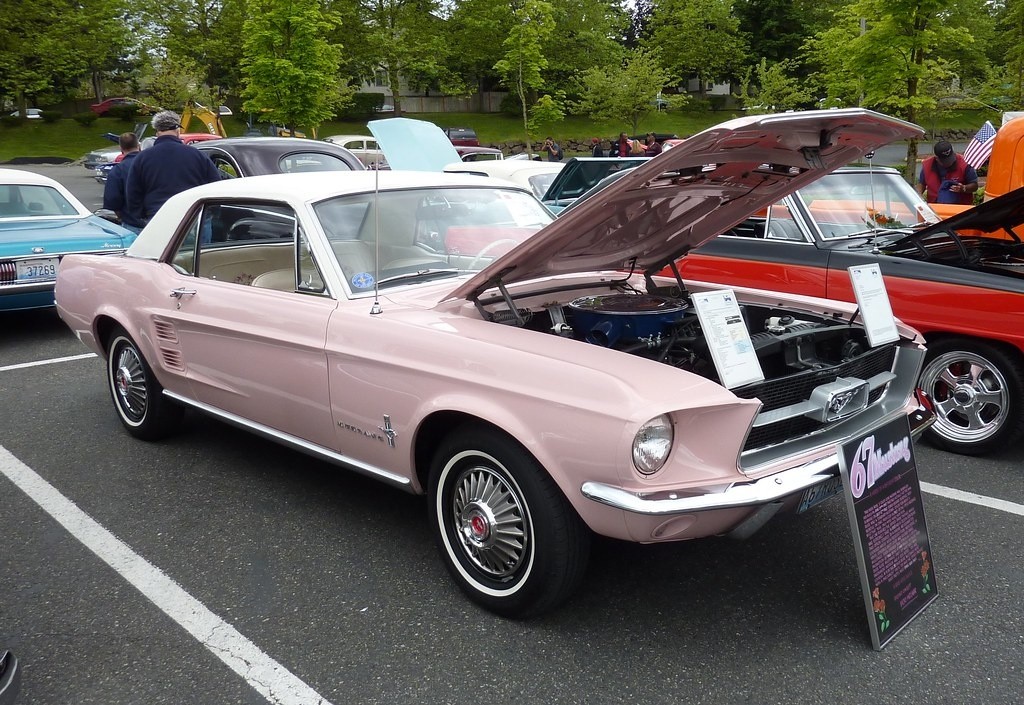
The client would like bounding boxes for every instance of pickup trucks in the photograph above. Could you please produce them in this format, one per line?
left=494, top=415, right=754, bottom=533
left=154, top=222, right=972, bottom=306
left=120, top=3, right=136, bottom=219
left=752, top=114, right=1024, bottom=244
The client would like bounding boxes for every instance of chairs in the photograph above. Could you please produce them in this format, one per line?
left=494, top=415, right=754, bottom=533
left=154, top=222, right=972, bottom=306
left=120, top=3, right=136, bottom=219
left=755, top=219, right=802, bottom=240
left=188, top=241, right=447, bottom=292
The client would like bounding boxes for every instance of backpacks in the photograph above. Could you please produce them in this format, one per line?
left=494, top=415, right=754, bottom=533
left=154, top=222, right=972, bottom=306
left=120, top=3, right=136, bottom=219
left=556, top=149, right=563, bottom=160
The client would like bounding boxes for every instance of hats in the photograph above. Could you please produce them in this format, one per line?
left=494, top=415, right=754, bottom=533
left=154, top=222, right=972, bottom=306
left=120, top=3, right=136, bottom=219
left=592, top=138, right=600, bottom=143
left=647, top=132, right=656, bottom=137
left=934, top=141, right=956, bottom=168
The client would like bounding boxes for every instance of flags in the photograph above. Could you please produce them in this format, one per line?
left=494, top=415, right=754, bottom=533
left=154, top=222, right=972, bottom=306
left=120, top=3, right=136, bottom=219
left=963, top=120, right=997, bottom=170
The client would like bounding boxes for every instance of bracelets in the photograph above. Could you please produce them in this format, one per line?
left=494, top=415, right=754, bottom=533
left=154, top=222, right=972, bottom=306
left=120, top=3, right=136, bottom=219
left=963, top=185, right=966, bottom=192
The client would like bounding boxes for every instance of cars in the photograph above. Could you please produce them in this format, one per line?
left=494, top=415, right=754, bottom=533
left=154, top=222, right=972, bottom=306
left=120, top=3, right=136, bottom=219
left=52, top=106, right=939, bottom=623
left=203, top=104, right=233, bottom=116
left=10, top=109, right=44, bottom=118
left=445, top=163, right=1024, bottom=455
left=87, top=116, right=656, bottom=263
left=375, top=104, right=407, bottom=113
left=90, top=97, right=164, bottom=116
left=0, top=168, right=138, bottom=313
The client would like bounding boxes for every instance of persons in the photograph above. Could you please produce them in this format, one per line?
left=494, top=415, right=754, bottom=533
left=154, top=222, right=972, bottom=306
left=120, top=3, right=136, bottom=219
left=127, top=110, right=222, bottom=246
left=103, top=132, right=141, bottom=225
left=612, top=132, right=663, bottom=157
left=541, top=137, right=560, bottom=162
left=918, top=141, right=978, bottom=205
left=592, top=138, right=603, bottom=157
